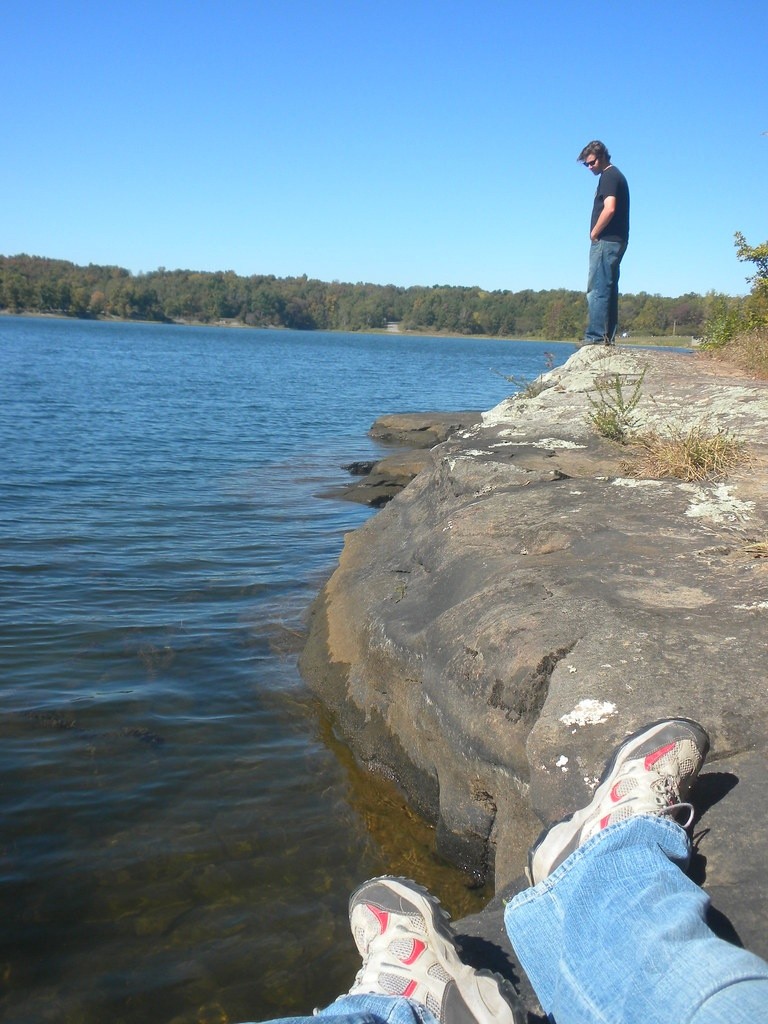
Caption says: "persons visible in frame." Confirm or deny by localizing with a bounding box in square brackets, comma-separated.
[575, 141, 630, 348]
[239, 717, 767, 1024]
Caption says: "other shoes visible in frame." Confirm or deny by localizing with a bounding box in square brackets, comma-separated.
[575, 338, 614, 349]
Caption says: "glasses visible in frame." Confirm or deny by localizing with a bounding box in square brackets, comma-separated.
[584, 155, 600, 167]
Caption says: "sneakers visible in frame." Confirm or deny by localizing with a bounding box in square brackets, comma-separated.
[348, 876, 525, 1024]
[525, 719, 709, 886]
